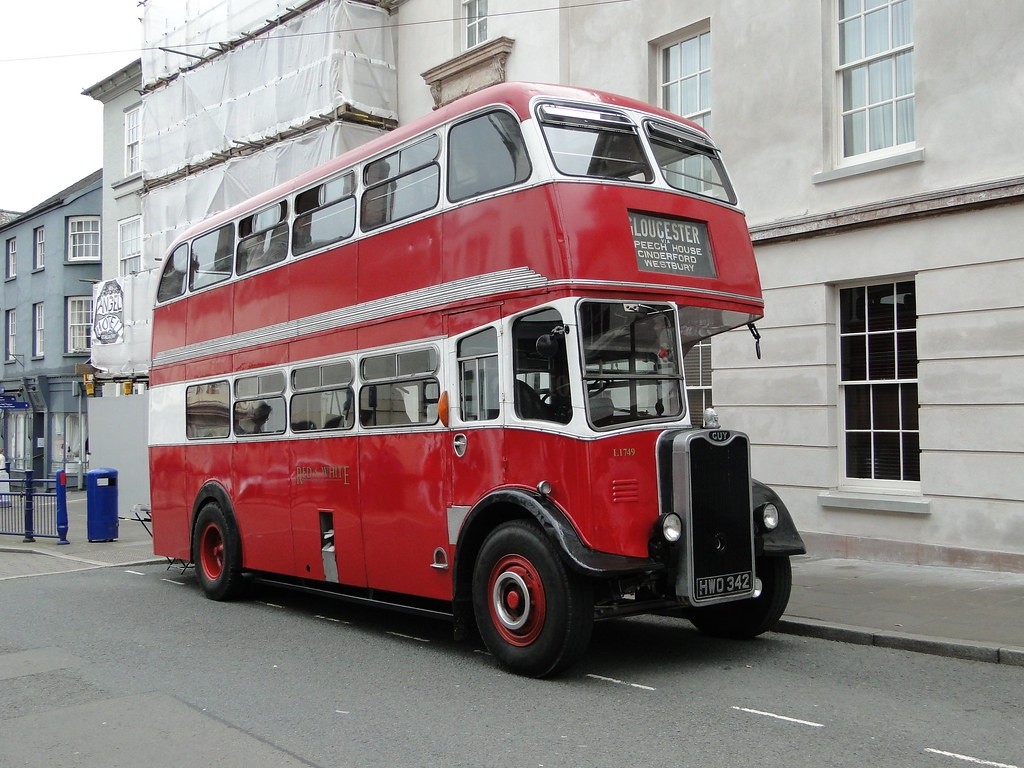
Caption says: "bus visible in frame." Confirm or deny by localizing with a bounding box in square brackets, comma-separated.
[147, 83, 808, 679]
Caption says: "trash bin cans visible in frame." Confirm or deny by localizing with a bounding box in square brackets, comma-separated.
[87, 467, 118, 542]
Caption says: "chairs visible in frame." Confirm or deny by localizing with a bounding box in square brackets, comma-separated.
[517, 378, 552, 420]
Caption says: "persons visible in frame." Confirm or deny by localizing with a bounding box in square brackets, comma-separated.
[240, 401, 272, 432]
[486, 363, 551, 421]
[0, 448, 12, 508]
